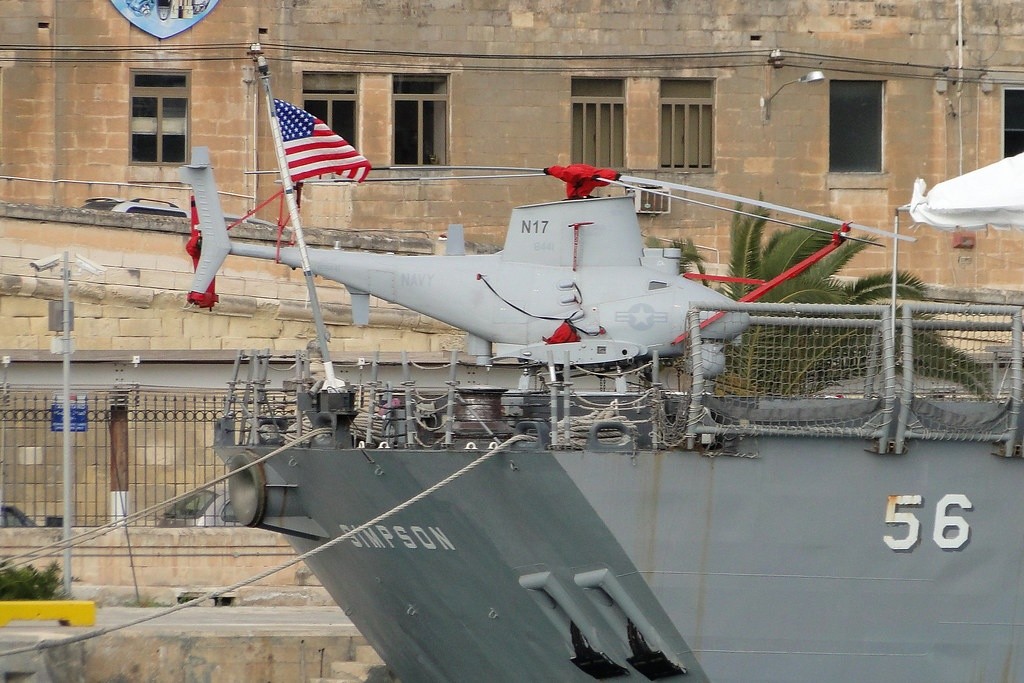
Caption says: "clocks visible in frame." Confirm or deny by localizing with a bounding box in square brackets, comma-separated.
[760, 67, 827, 121]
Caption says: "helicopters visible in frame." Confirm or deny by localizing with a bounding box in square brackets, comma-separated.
[175, 92, 912, 397]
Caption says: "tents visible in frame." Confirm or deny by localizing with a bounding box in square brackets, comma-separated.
[886, 147, 1024, 360]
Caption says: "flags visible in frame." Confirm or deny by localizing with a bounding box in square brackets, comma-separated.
[267, 94, 373, 191]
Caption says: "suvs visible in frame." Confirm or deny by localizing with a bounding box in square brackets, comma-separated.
[79, 195, 191, 218]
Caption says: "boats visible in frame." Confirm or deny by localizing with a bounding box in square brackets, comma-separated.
[209, 325, 1024, 683]
